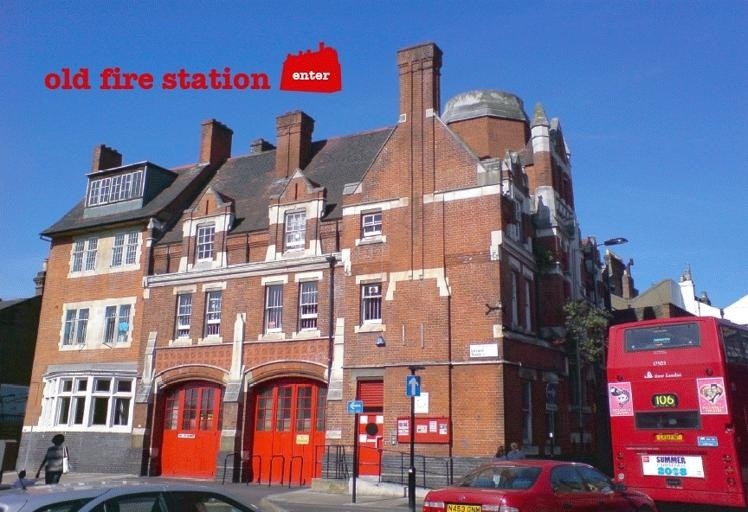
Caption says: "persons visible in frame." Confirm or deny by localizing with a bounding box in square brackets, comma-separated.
[492, 446, 506, 485]
[507, 442, 526, 460]
[36, 433, 69, 484]
[11, 470, 34, 489]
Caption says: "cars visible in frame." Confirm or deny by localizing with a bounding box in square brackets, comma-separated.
[423, 459, 657, 512]
[0, 476, 283, 512]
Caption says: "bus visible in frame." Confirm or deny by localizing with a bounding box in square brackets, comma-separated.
[606, 315, 747, 509]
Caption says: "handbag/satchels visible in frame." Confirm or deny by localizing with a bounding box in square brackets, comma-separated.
[62, 447, 70, 474]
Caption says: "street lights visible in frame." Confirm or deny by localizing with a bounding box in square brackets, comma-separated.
[572, 236, 627, 459]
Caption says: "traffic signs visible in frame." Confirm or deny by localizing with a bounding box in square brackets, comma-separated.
[545, 404, 558, 411]
